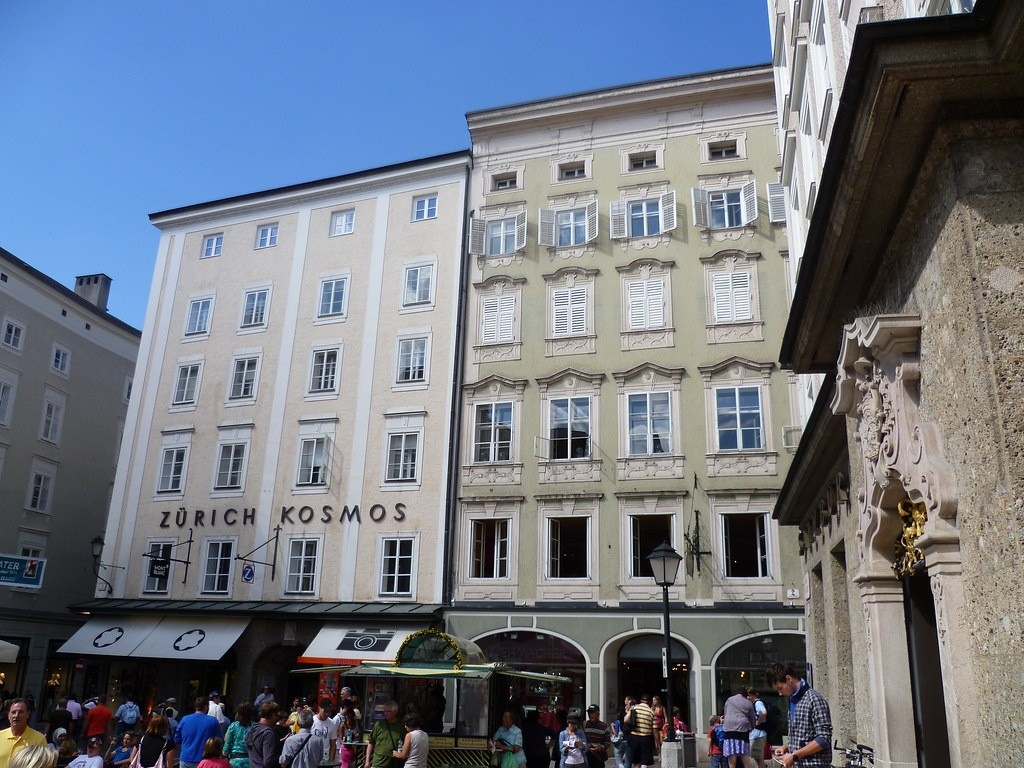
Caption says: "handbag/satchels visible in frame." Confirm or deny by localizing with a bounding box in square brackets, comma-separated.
[609, 720, 624, 745]
[390, 749, 403, 768]
[267, 757, 292, 768]
[500, 746, 519, 768]
[754, 721, 766, 730]
[628, 704, 637, 729]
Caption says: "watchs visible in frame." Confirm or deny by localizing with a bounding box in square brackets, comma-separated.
[793, 752, 799, 762]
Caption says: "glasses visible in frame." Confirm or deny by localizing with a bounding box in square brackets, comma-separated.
[568, 720, 575, 724]
[59, 734, 66, 737]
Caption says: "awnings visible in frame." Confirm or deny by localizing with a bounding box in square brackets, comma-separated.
[295, 621, 432, 666]
[56, 615, 254, 662]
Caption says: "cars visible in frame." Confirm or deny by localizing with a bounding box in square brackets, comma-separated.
[522, 695, 556, 718]
[555, 699, 585, 718]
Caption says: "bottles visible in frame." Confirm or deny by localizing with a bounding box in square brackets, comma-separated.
[347, 728, 350, 742]
[398, 739, 403, 753]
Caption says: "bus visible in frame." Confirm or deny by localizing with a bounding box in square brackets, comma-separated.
[716, 667, 806, 718]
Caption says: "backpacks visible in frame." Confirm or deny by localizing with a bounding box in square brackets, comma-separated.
[130, 735, 168, 768]
[125, 704, 137, 725]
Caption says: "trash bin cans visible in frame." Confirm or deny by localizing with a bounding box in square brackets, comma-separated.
[676, 732, 696, 768]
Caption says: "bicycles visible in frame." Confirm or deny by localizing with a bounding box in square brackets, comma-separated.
[834, 740, 874, 768]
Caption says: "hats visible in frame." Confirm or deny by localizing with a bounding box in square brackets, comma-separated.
[320, 699, 333, 712]
[53, 728, 67, 742]
[566, 712, 578, 724]
[88, 737, 102, 746]
[213, 691, 220, 696]
[587, 704, 599, 712]
[167, 697, 176, 703]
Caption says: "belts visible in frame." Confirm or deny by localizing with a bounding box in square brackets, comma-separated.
[230, 753, 250, 758]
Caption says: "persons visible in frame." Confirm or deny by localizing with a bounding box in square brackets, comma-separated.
[0, 659, 831, 768]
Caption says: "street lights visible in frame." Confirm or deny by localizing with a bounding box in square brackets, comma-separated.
[646, 540, 683, 768]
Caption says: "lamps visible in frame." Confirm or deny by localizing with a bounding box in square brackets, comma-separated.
[89, 535, 125, 595]
